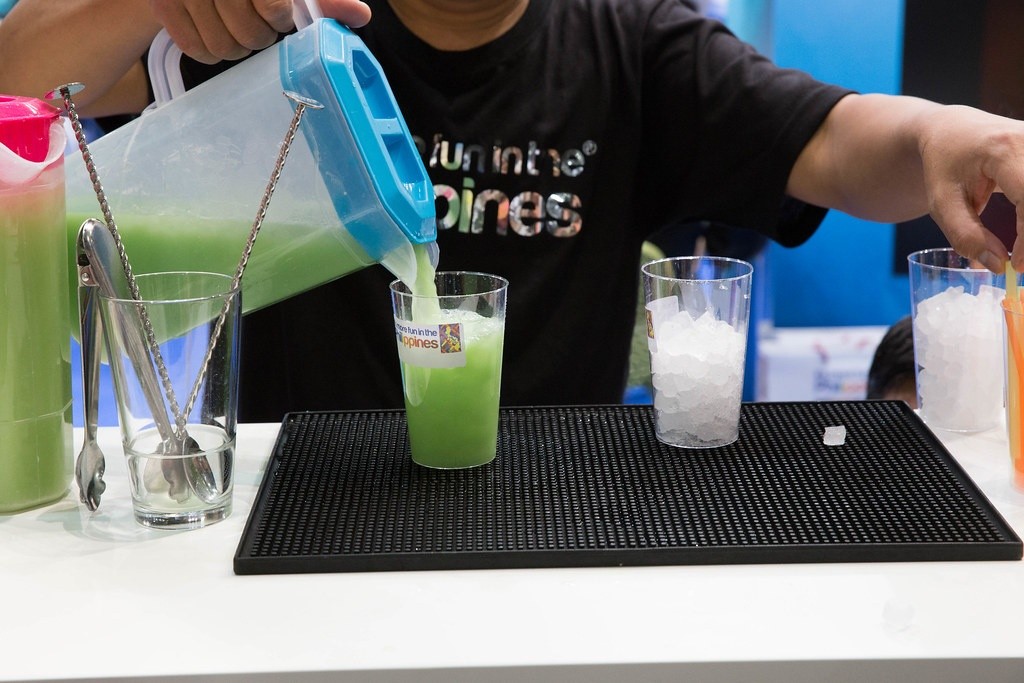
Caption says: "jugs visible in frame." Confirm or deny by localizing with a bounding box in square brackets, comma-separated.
[0, 93, 70, 517]
[64, 1, 439, 369]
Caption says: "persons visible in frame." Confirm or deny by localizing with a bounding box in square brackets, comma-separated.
[0, 0, 1024, 424]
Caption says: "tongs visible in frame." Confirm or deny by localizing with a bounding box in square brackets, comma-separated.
[74, 217, 194, 513]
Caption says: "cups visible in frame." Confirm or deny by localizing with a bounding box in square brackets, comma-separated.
[1000, 285, 1024, 492]
[390, 270, 508, 470]
[906, 247, 1013, 434]
[96, 271, 244, 532]
[640, 255, 754, 450]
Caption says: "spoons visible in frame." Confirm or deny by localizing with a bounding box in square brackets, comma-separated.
[42, 81, 221, 507]
[143, 89, 326, 490]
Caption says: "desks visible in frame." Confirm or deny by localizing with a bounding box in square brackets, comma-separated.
[0, 409, 1024, 683]
